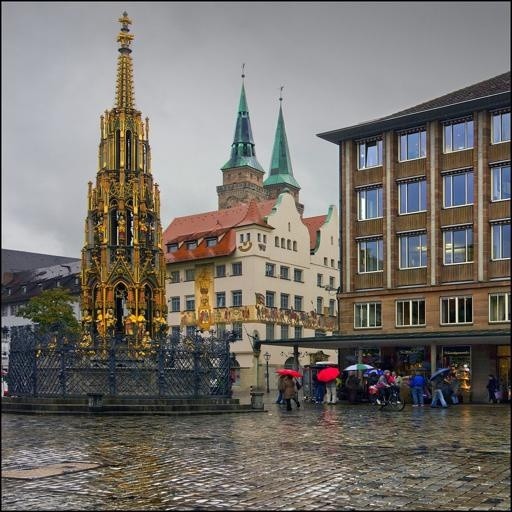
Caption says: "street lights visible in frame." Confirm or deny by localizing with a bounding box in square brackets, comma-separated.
[262, 351, 272, 393]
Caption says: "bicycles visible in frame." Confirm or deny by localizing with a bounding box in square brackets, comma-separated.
[369, 382, 407, 411]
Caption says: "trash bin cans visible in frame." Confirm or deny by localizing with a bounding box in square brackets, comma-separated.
[250, 392, 264, 409]
[87, 392, 104, 412]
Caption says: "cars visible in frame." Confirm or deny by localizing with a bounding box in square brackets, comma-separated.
[1, 369, 32, 397]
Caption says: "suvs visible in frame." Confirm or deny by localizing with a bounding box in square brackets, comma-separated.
[167, 356, 222, 391]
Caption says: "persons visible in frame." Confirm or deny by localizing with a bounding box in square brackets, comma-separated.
[486, 374, 497, 403]
[183, 305, 324, 330]
[49, 306, 165, 357]
[92, 216, 148, 245]
[276, 369, 459, 409]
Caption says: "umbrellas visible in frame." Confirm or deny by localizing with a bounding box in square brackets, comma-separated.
[316, 362, 383, 382]
